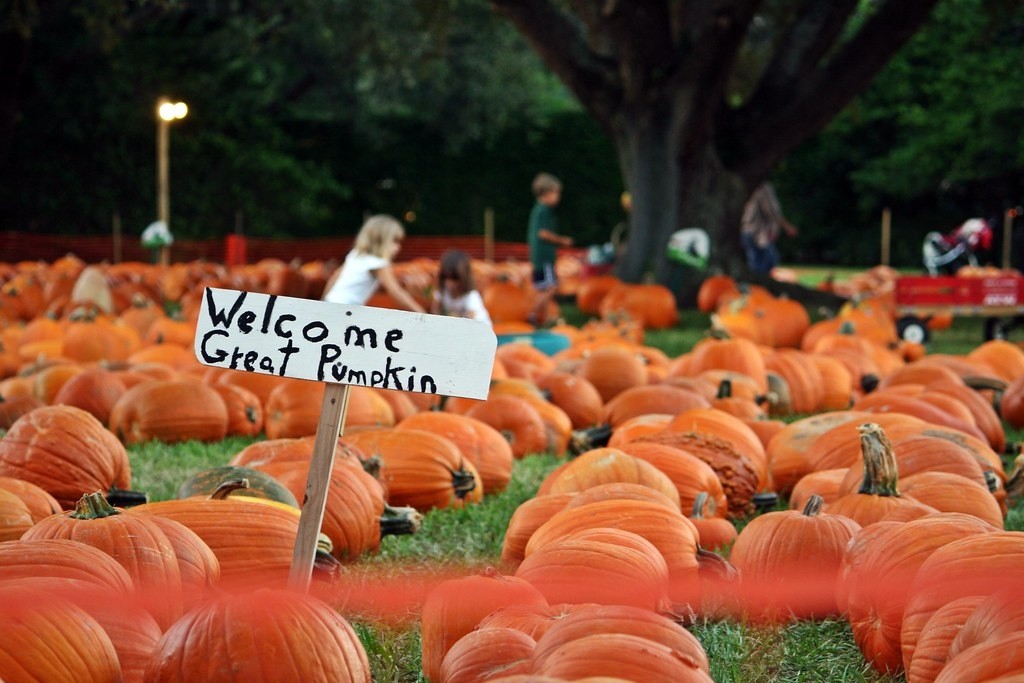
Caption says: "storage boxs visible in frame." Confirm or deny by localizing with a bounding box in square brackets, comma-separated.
[897, 274, 1024, 306]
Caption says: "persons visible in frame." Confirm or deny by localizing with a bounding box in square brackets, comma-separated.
[140, 216, 172, 259]
[528, 174, 573, 291]
[432, 246, 490, 324]
[923, 224, 970, 280]
[663, 218, 710, 299]
[327, 215, 428, 315]
[742, 172, 796, 288]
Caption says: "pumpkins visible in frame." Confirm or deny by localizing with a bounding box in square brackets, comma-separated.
[0, 261, 1024, 683]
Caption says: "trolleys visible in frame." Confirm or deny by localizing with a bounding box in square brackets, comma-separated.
[891, 260, 1024, 345]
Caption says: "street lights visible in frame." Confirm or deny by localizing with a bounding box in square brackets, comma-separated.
[153, 96, 188, 264]
